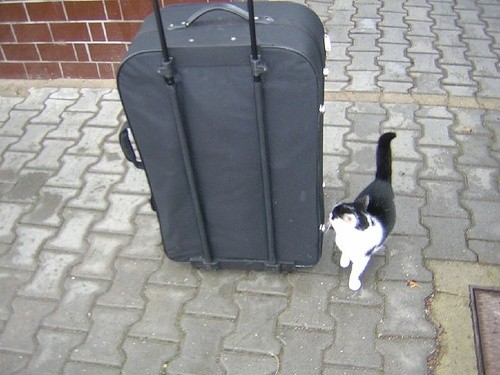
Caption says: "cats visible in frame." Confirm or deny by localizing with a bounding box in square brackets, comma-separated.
[329, 132, 396, 291]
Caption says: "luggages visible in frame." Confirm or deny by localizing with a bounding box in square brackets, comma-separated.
[116, 0, 332, 273]
